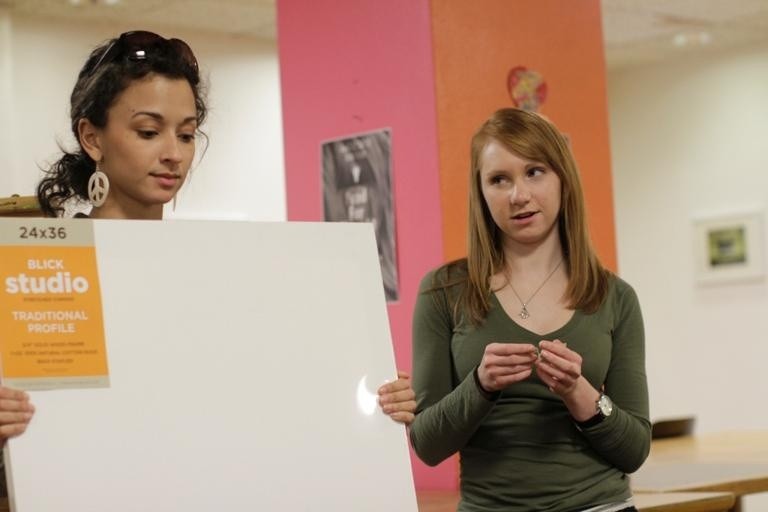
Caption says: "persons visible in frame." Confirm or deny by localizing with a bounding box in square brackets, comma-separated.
[0, 28, 416, 440]
[409, 107, 653, 512]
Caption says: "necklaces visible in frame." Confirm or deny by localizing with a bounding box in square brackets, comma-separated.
[498, 256, 567, 319]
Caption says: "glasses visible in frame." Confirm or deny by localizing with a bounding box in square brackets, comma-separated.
[88, 30, 200, 81]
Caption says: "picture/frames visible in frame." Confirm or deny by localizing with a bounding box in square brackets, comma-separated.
[692, 211, 761, 283]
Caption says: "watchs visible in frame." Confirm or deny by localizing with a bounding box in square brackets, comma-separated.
[569, 388, 613, 433]
